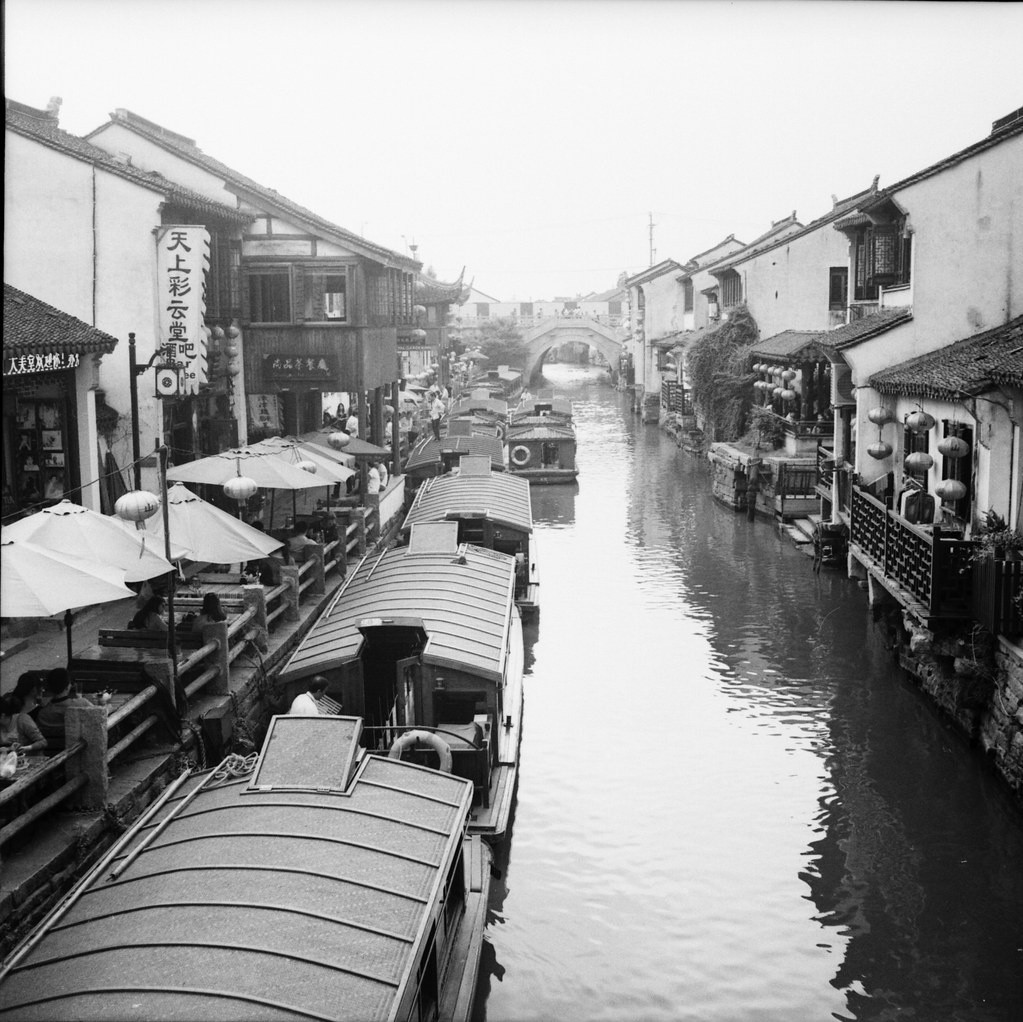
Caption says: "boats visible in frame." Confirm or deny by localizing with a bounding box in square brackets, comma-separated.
[404, 366, 580, 622]
[276, 521, 525, 855]
[0, 715, 492, 1022]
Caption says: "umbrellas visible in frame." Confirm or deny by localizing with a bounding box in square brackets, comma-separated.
[1, 481, 286, 669]
[105, 450, 128, 514]
[156, 426, 388, 521]
[386, 382, 429, 402]
[458, 351, 489, 359]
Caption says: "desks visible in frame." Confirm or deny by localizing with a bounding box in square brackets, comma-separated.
[321, 506, 353, 517]
[4, 755, 49, 781]
[74, 692, 136, 716]
[914, 522, 963, 541]
[72, 644, 198, 666]
[159, 611, 244, 626]
[176, 583, 275, 600]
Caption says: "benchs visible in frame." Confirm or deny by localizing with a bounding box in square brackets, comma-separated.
[67, 658, 154, 695]
[198, 572, 240, 587]
[162, 597, 244, 646]
[97, 629, 202, 676]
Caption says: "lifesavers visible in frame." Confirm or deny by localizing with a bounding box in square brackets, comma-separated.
[387, 729, 453, 775]
[511, 445, 531, 466]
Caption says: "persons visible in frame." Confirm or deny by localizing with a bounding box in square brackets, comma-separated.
[20, 405, 59, 466]
[1, 672, 96, 815]
[130, 364, 470, 633]
[511, 307, 600, 322]
[763, 390, 834, 433]
[291, 676, 328, 716]
[1, 476, 63, 516]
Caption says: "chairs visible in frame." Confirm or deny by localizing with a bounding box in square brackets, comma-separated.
[905, 489, 936, 525]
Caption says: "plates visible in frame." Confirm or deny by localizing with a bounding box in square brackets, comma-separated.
[16, 762, 29, 770]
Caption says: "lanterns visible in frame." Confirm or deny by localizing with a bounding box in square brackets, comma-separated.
[114, 432, 350, 531]
[867, 406, 968, 501]
[403, 352, 456, 380]
[412, 281, 463, 341]
[621, 290, 676, 370]
[211, 325, 240, 376]
[753, 363, 796, 400]
